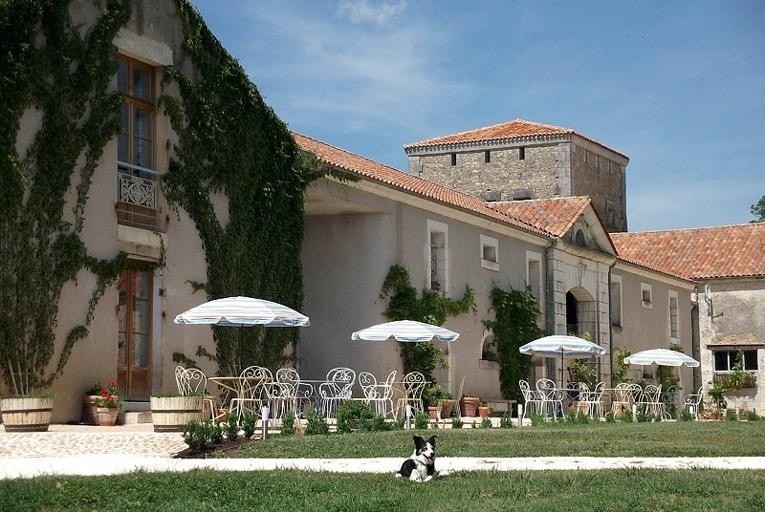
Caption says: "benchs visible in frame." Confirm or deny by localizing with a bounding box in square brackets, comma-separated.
[483, 399, 517, 418]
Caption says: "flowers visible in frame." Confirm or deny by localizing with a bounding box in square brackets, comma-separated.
[96, 394, 122, 410]
[86, 379, 121, 396]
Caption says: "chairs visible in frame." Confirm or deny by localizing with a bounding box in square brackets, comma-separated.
[176, 366, 426, 426]
[518, 378, 705, 421]
[437, 375, 465, 421]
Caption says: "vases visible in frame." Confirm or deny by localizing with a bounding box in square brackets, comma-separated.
[84, 395, 119, 424]
[97, 408, 120, 426]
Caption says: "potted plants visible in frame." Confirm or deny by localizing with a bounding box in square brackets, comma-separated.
[150, 392, 204, 432]
[427, 383, 492, 419]
[0, 391, 57, 433]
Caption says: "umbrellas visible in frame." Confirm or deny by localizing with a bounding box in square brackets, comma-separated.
[623, 348, 701, 392]
[519, 335, 607, 388]
[351, 320, 462, 381]
[174, 295, 311, 375]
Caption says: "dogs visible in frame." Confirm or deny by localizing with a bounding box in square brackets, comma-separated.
[393, 433, 449, 485]
